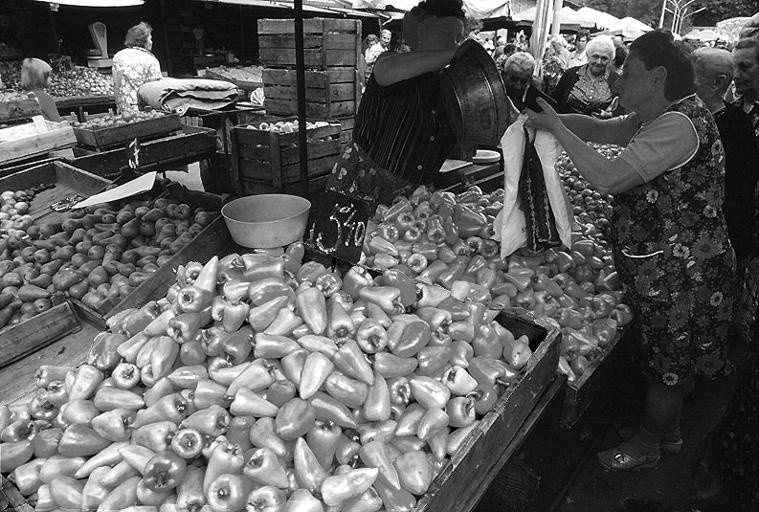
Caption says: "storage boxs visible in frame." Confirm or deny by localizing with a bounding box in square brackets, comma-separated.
[233, 14, 365, 201]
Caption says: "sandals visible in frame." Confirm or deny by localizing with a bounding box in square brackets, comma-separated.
[597, 438, 684, 473]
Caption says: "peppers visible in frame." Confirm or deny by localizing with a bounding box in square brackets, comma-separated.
[0, 185, 633, 512]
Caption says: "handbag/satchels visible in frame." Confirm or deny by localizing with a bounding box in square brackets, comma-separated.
[517, 123, 563, 255]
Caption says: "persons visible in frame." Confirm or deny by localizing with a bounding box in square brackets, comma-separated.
[678, 34, 758, 354]
[110, 19, 164, 116]
[525, 30, 735, 472]
[304, 2, 628, 277]
[18, 56, 62, 122]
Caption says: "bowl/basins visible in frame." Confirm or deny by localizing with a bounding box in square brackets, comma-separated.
[438, 38, 508, 149]
[222, 193, 311, 249]
